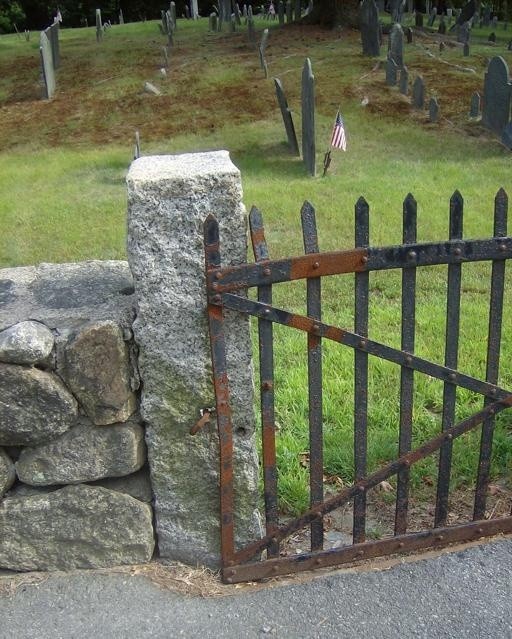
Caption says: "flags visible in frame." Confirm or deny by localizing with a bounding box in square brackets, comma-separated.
[331, 113, 346, 151]
